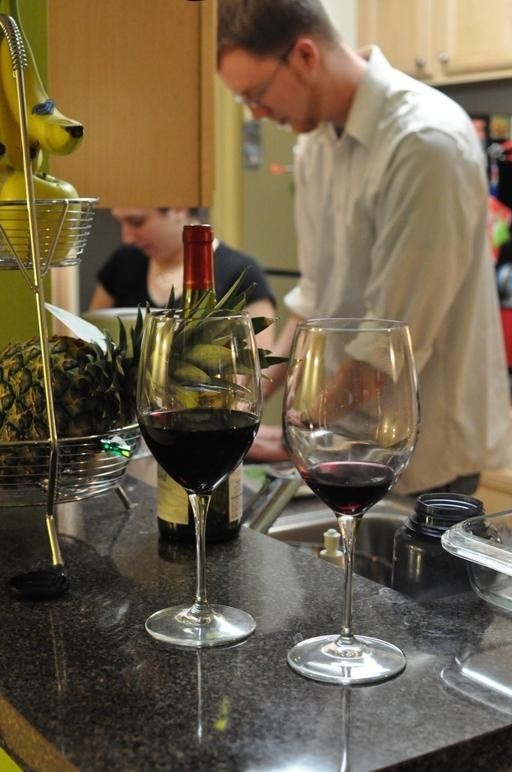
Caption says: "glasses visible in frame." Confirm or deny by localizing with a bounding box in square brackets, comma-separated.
[235, 61, 287, 107]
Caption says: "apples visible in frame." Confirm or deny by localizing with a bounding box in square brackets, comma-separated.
[0, 169, 82, 264]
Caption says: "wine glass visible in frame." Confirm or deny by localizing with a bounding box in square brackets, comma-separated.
[134, 306, 267, 647]
[279, 316, 421, 687]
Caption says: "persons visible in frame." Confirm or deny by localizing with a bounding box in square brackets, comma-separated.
[176, 0, 511, 521]
[87, 206, 278, 398]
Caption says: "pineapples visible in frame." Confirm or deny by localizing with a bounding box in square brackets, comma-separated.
[0, 266, 292, 491]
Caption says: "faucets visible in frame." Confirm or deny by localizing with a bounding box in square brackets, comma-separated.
[238, 462, 309, 532]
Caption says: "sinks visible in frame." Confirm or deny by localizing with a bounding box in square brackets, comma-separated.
[242, 500, 510, 599]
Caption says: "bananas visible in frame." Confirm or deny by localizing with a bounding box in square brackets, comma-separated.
[0, 19, 84, 177]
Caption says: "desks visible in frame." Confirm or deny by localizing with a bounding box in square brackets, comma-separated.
[0, 474, 512, 772]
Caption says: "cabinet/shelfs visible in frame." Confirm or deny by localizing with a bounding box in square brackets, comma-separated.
[354, 0, 512, 88]
[47, 0, 216, 206]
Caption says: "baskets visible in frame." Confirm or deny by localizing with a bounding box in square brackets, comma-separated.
[0, 423, 141, 506]
[0, 197, 97, 271]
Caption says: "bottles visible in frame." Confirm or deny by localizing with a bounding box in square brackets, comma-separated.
[392, 489, 504, 644]
[151, 219, 245, 546]
